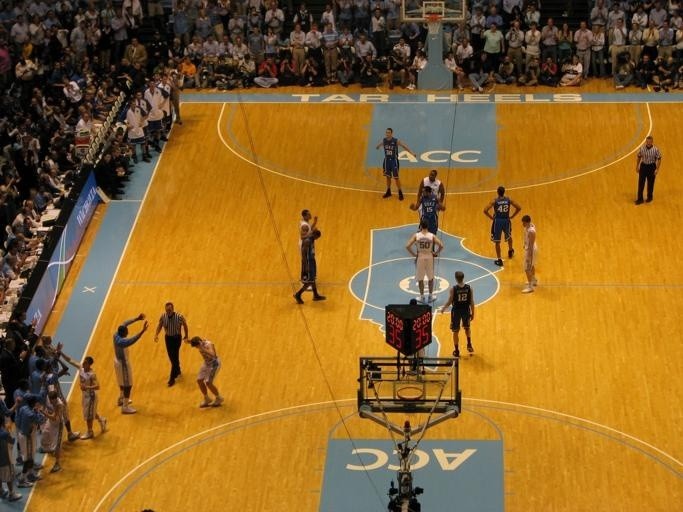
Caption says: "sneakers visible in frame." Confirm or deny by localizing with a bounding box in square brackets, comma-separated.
[168, 378, 175, 385]
[427, 295, 437, 303]
[16, 479, 34, 489]
[8, 492, 22, 501]
[526, 280, 537, 286]
[117, 397, 132, 406]
[520, 287, 534, 293]
[383, 192, 391, 198]
[398, 192, 404, 200]
[467, 344, 473, 353]
[199, 397, 212, 408]
[494, 259, 503, 267]
[67, 431, 81, 441]
[207, 396, 224, 406]
[312, 295, 326, 301]
[507, 248, 515, 258]
[100, 418, 106, 433]
[50, 463, 63, 474]
[80, 431, 94, 439]
[452, 350, 459, 356]
[122, 406, 137, 414]
[292, 293, 305, 305]
[414, 297, 425, 303]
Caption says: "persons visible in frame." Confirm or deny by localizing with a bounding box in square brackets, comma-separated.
[440, 271, 477, 356]
[64, 355, 107, 439]
[419, 171, 444, 212]
[405, 218, 444, 304]
[410, 185, 444, 236]
[483, 187, 521, 266]
[112, 314, 149, 415]
[374, 128, 417, 201]
[154, 304, 189, 386]
[520, 215, 537, 293]
[442, 1, 683, 91]
[189, 336, 223, 409]
[0, 0, 181, 202]
[2, 202, 79, 503]
[407, 298, 425, 373]
[292, 230, 326, 304]
[636, 136, 662, 206]
[171, 1, 427, 92]
[300, 210, 316, 244]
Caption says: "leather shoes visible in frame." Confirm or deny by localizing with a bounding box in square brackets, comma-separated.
[646, 198, 652, 202]
[634, 199, 644, 205]
